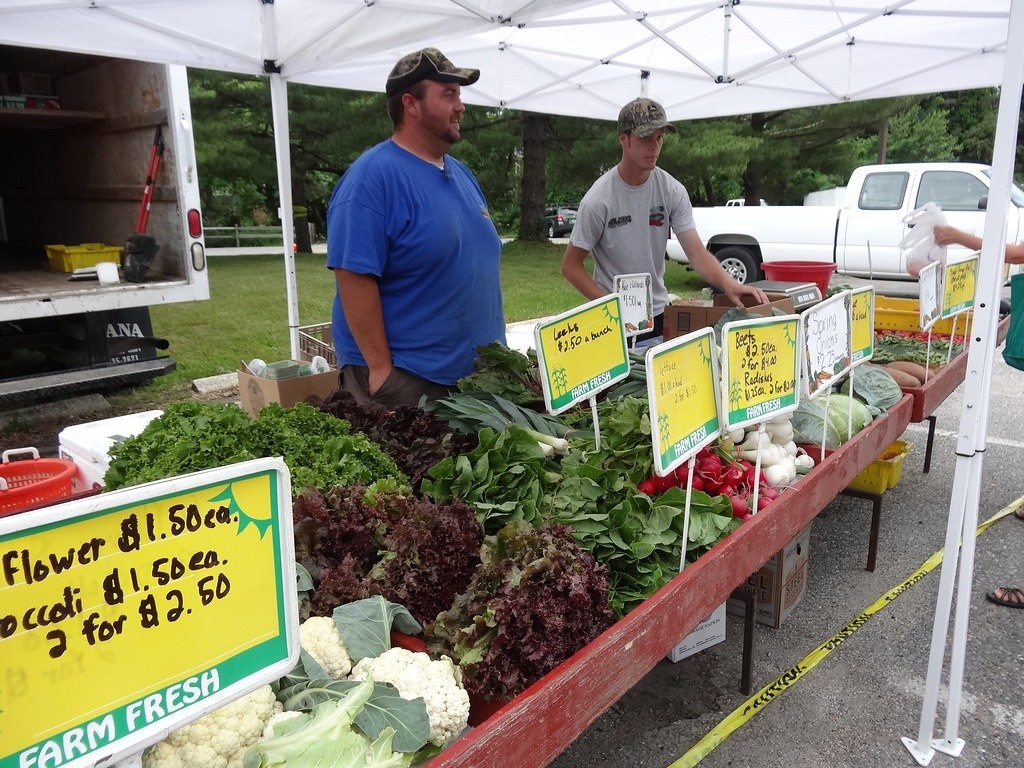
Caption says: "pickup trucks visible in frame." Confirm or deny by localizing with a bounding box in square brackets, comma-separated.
[726, 198, 767, 208]
[660, 163, 1024, 298]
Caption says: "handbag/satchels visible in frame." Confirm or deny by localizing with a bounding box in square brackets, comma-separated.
[899, 201, 948, 276]
[1001, 273, 1024, 371]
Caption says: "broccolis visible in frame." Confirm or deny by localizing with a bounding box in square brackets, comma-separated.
[138, 614, 469, 768]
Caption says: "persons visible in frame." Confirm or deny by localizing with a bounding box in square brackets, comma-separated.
[933, 226, 1024, 609]
[561, 99, 770, 348]
[323, 47, 508, 409]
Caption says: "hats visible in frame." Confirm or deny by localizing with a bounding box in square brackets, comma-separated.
[386, 47, 481, 97]
[618, 97, 677, 138]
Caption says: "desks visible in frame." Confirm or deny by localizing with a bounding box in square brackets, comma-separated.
[424, 306, 1015, 767]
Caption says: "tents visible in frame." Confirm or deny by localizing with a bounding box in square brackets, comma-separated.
[0, 0, 1024, 768]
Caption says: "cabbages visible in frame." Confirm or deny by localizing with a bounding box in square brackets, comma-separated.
[790, 363, 904, 450]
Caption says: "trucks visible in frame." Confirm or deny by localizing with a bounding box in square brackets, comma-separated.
[0, 44, 216, 410]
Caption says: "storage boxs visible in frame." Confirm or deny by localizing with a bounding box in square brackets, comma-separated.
[663, 292, 794, 350]
[56, 406, 166, 494]
[667, 601, 725, 664]
[847, 439, 911, 494]
[727, 519, 815, 627]
[43, 242, 124, 272]
[0, 71, 61, 110]
[236, 319, 334, 415]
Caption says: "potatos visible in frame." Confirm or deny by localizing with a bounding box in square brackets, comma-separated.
[880, 361, 935, 387]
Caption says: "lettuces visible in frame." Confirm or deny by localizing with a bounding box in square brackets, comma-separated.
[100, 396, 615, 700]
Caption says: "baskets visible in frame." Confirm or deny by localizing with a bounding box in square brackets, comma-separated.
[0, 447, 76, 516]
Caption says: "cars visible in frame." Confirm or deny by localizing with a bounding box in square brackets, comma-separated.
[545, 206, 579, 239]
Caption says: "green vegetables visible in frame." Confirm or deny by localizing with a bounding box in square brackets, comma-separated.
[302, 339, 749, 619]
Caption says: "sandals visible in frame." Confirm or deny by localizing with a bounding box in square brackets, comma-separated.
[1015, 507, 1024, 519]
[987, 585, 1024, 608]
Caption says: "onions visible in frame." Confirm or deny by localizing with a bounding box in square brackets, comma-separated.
[724, 410, 815, 492]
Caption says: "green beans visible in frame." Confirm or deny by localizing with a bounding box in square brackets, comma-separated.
[870, 334, 964, 364]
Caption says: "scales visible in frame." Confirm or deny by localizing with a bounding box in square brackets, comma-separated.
[741, 280, 823, 312]
